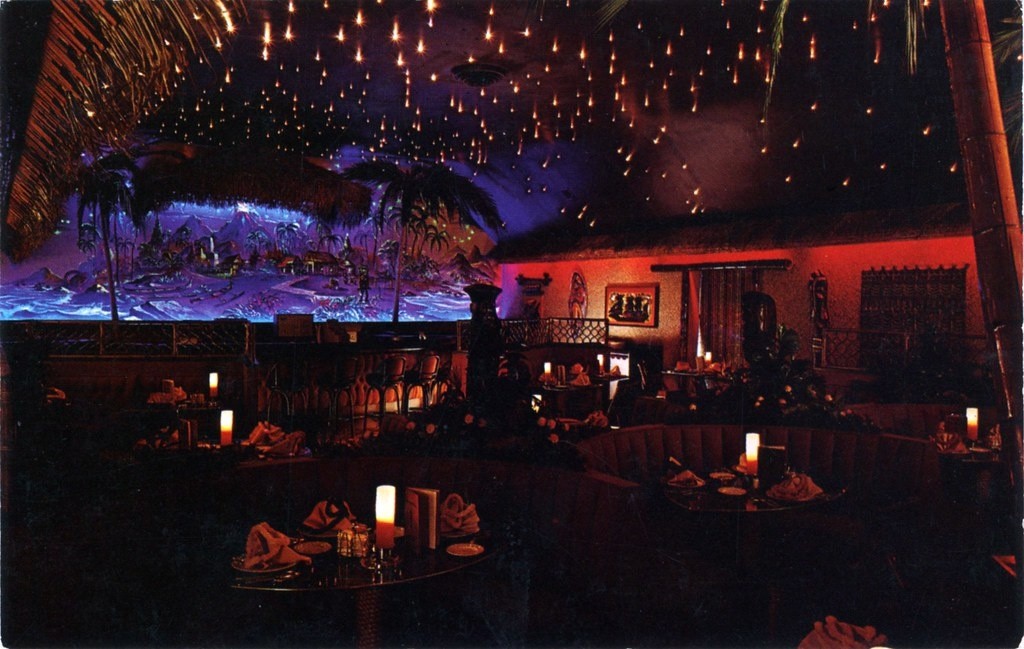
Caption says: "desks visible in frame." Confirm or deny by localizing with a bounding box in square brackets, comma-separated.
[662, 370, 716, 413]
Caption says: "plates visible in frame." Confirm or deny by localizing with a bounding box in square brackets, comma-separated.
[232, 553, 296, 572]
[447, 543, 483, 556]
[718, 487, 746, 495]
[440, 530, 479, 538]
[798, 624, 890, 649]
[767, 489, 816, 501]
[296, 525, 337, 537]
[936, 449, 969, 454]
[667, 479, 705, 487]
[709, 472, 735, 480]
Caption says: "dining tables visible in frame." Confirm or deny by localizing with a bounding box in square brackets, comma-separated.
[660, 472, 851, 648]
[956, 455, 1004, 506]
[221, 531, 505, 649]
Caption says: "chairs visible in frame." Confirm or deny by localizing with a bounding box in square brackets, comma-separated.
[266, 352, 465, 439]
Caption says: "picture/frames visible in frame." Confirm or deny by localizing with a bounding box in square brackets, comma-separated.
[605, 286, 658, 329]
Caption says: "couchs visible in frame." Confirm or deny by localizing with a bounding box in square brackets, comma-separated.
[840, 402, 1005, 530]
[567, 425, 942, 629]
[232, 456, 649, 648]
